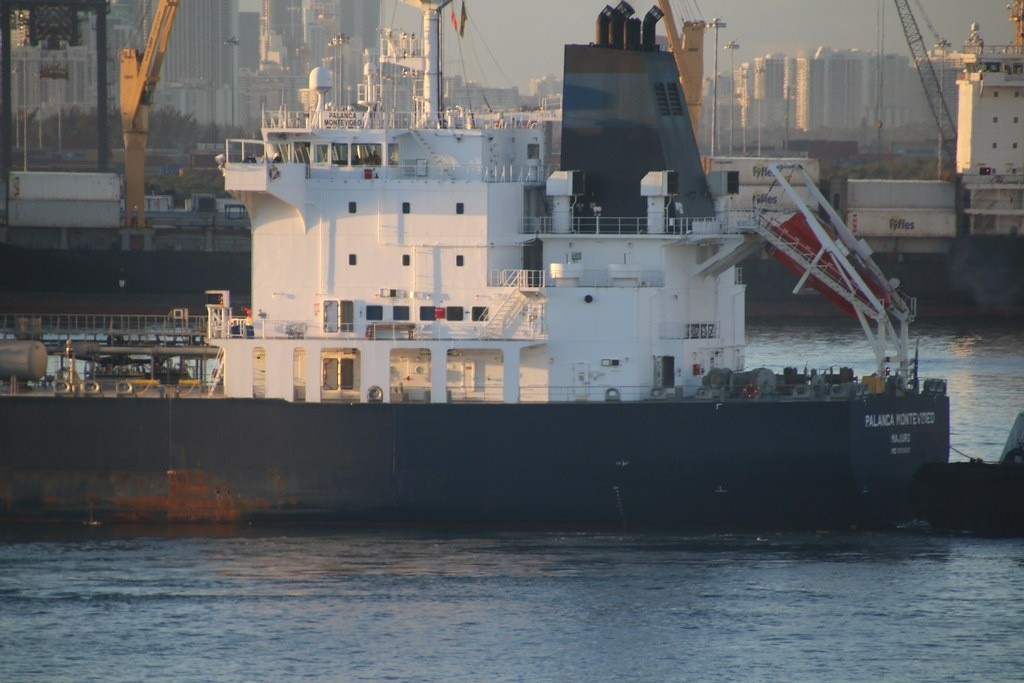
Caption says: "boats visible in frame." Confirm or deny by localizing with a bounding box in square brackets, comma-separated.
[0, 0, 949, 536]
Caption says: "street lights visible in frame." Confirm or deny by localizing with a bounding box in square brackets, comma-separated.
[327, 33, 349, 110]
[722, 39, 739, 157]
[704, 17, 727, 157]
[738, 57, 766, 156]
[224, 36, 239, 139]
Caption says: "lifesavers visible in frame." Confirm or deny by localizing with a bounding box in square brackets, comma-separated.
[742, 383, 758, 398]
